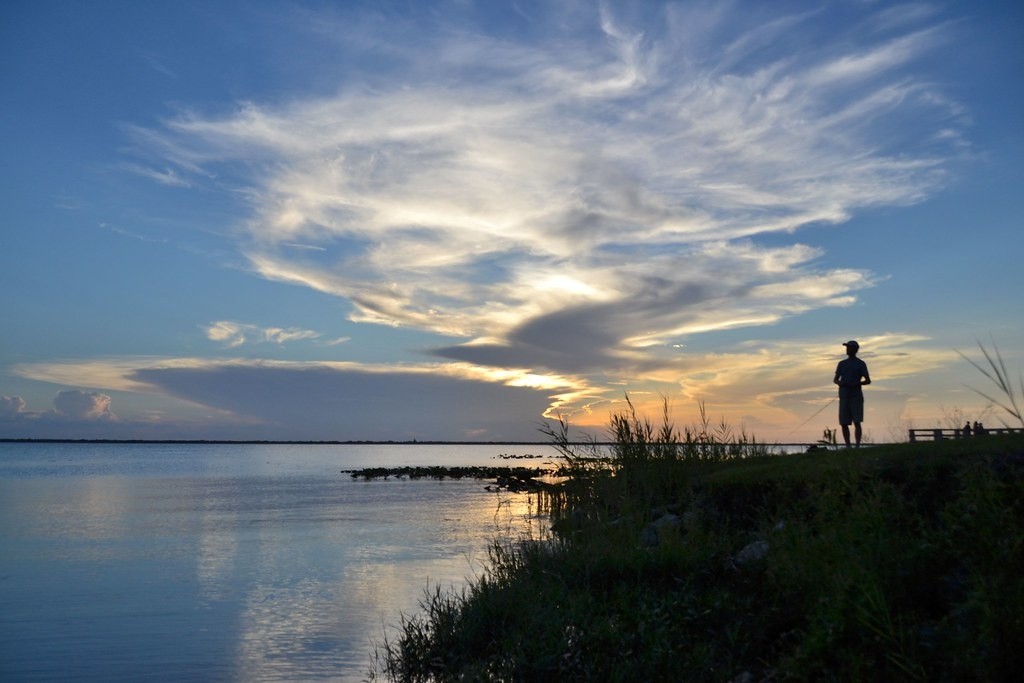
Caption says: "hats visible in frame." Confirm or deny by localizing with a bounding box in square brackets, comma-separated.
[843, 341, 859, 348]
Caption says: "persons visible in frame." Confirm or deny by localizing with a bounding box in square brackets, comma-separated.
[834, 340, 872, 446]
[964, 421, 971, 436]
[974, 421, 983, 435]
